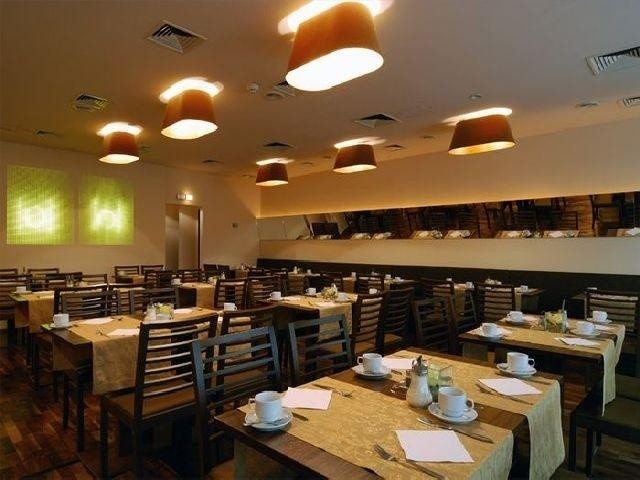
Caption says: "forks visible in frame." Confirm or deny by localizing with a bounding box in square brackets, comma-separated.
[372, 442, 447, 480]
[310, 382, 354, 398]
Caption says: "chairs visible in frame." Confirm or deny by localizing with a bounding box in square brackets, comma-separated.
[1, 261, 640, 480]
[310, 192, 638, 241]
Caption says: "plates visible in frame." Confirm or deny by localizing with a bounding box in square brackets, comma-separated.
[424, 402, 480, 424]
[245, 409, 291, 432]
[9, 291, 32, 294]
[478, 333, 502, 340]
[49, 323, 72, 329]
[496, 361, 538, 378]
[171, 283, 183, 286]
[351, 363, 392, 380]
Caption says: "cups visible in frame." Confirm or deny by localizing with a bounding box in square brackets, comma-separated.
[16, 286, 27, 293]
[438, 386, 474, 418]
[357, 352, 383, 372]
[248, 390, 282, 424]
[53, 314, 69, 326]
[384, 274, 528, 291]
[223, 302, 237, 311]
[174, 279, 180, 284]
[480, 310, 608, 333]
[271, 287, 348, 301]
[506, 352, 535, 371]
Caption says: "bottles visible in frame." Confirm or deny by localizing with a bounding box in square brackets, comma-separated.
[147, 304, 156, 321]
[405, 356, 433, 407]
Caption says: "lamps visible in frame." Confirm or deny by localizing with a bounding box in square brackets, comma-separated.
[157, 75, 225, 141]
[251, 155, 296, 188]
[95, 121, 146, 166]
[276, 0, 395, 94]
[441, 106, 518, 157]
[331, 133, 388, 178]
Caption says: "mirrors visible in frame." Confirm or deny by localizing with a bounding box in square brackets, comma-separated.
[256, 191, 640, 242]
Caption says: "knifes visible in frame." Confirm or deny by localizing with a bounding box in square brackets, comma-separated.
[416, 416, 493, 445]
